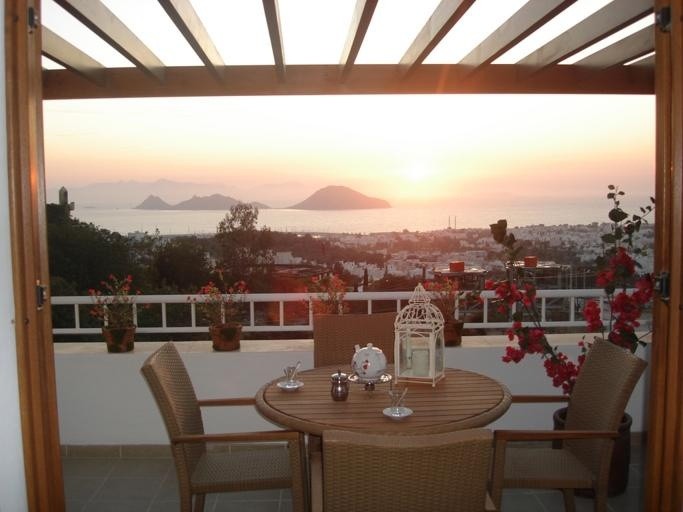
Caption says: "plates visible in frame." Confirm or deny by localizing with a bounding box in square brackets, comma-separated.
[276, 380, 304, 391]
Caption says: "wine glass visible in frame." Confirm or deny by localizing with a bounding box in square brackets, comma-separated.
[387, 386, 408, 415]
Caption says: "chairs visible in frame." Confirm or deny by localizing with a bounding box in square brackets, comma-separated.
[141, 342, 307, 512]
[487, 337, 648, 511]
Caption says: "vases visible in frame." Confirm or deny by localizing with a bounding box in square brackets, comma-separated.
[209, 325, 242, 351]
[444, 321, 464, 347]
[553, 408, 632, 497]
[101, 325, 136, 353]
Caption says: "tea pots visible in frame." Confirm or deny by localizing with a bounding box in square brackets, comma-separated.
[352, 343, 386, 378]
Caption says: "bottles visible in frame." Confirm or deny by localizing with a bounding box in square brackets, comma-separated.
[330, 367, 350, 401]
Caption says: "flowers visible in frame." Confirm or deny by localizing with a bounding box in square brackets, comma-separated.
[86, 273, 151, 327]
[484, 186, 656, 401]
[299, 270, 350, 315]
[186, 280, 251, 325]
[423, 270, 473, 321]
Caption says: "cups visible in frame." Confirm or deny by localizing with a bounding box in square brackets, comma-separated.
[284, 366, 297, 386]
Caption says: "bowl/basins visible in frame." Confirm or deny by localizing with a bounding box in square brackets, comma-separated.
[382, 406, 413, 420]
